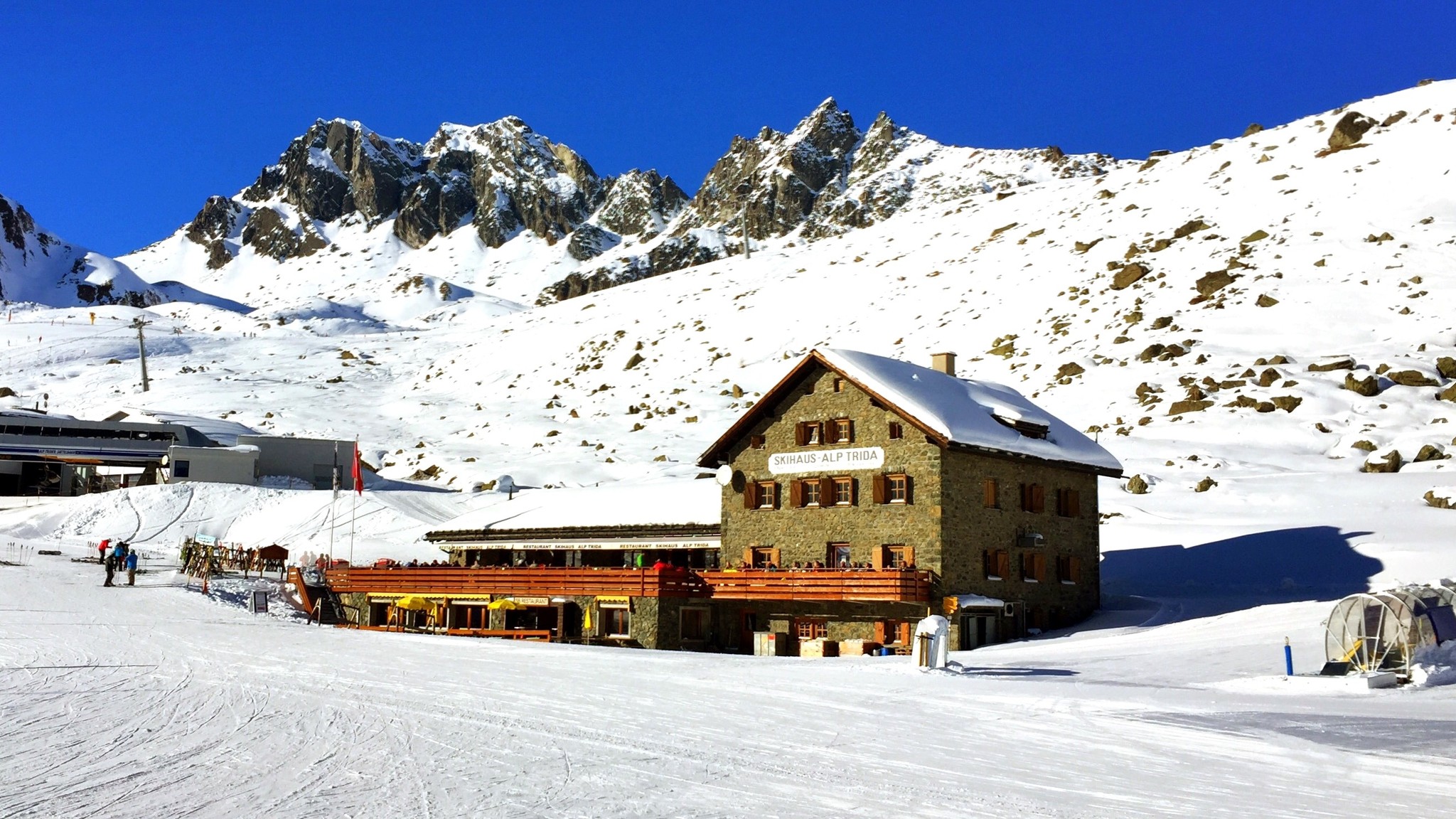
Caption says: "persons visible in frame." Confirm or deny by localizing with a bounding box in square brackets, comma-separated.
[789, 560, 822, 572]
[637, 551, 684, 571]
[299, 551, 309, 566]
[112, 541, 129, 572]
[566, 564, 629, 570]
[724, 561, 777, 572]
[372, 559, 461, 569]
[471, 560, 480, 570]
[125, 549, 138, 586]
[501, 559, 552, 570]
[885, 561, 919, 573]
[316, 553, 326, 570]
[839, 561, 876, 572]
[103, 552, 116, 587]
[98, 538, 112, 564]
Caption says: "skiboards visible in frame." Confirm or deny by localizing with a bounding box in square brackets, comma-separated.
[0, 536, 256, 588]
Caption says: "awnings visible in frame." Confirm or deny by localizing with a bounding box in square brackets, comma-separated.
[439, 536, 722, 555]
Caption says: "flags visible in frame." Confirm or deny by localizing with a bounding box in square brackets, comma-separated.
[351, 442, 363, 495]
[333, 450, 339, 499]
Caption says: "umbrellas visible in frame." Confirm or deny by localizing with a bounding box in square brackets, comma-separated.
[396, 596, 435, 612]
[487, 599, 526, 611]
[584, 602, 594, 644]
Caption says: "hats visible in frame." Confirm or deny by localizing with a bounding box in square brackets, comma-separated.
[108, 538, 112, 542]
[130, 549, 135, 554]
